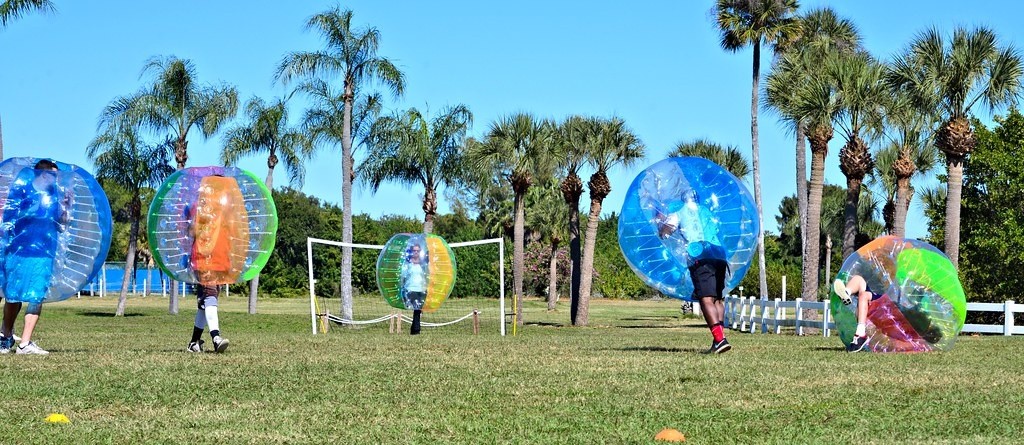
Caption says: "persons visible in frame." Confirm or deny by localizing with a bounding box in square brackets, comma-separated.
[400, 244, 430, 335]
[834, 252, 946, 353]
[0, 160, 76, 355]
[656, 189, 732, 355]
[183, 174, 229, 354]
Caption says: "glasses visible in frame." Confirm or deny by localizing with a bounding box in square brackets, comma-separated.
[46, 171, 57, 178]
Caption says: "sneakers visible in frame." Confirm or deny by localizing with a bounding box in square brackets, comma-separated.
[16, 341, 50, 356]
[213, 335, 230, 354]
[187, 340, 203, 353]
[0, 333, 15, 354]
[834, 279, 852, 305]
[848, 334, 869, 354]
[707, 338, 731, 354]
